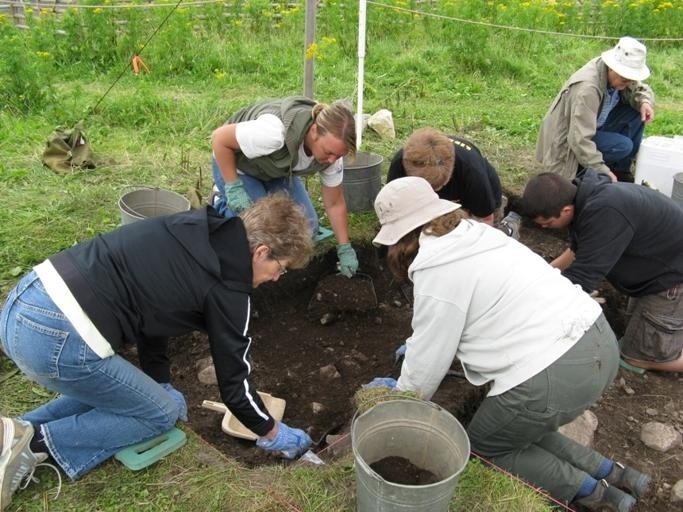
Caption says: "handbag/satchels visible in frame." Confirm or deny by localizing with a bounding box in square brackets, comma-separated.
[44, 119, 105, 178]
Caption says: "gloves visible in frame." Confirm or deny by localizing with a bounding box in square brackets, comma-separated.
[394, 343, 407, 362]
[364, 377, 396, 391]
[257, 420, 313, 460]
[223, 180, 255, 216]
[335, 242, 360, 278]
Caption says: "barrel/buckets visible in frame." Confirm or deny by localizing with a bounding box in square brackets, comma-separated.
[633, 134, 683, 198]
[117, 184, 192, 226]
[670, 172, 683, 204]
[350, 394, 472, 512]
[339, 150, 384, 212]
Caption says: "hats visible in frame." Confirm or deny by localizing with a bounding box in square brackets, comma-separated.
[372, 176, 463, 249]
[601, 36, 651, 82]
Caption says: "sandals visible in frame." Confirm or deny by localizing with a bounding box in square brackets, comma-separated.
[207, 181, 222, 205]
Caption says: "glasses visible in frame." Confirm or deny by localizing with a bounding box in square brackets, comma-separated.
[273, 256, 288, 276]
[620, 77, 632, 83]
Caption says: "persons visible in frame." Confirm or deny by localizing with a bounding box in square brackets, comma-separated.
[370, 176, 652, 511]
[211, 97, 359, 280]
[534, 37, 655, 183]
[522, 172, 683, 371]
[377, 125, 524, 269]
[0, 191, 313, 511]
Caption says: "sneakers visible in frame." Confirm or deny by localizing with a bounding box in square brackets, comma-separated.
[1, 417, 61, 512]
[604, 460, 653, 497]
[572, 479, 636, 512]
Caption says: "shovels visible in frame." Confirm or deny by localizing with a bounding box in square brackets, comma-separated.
[271, 422, 345, 456]
